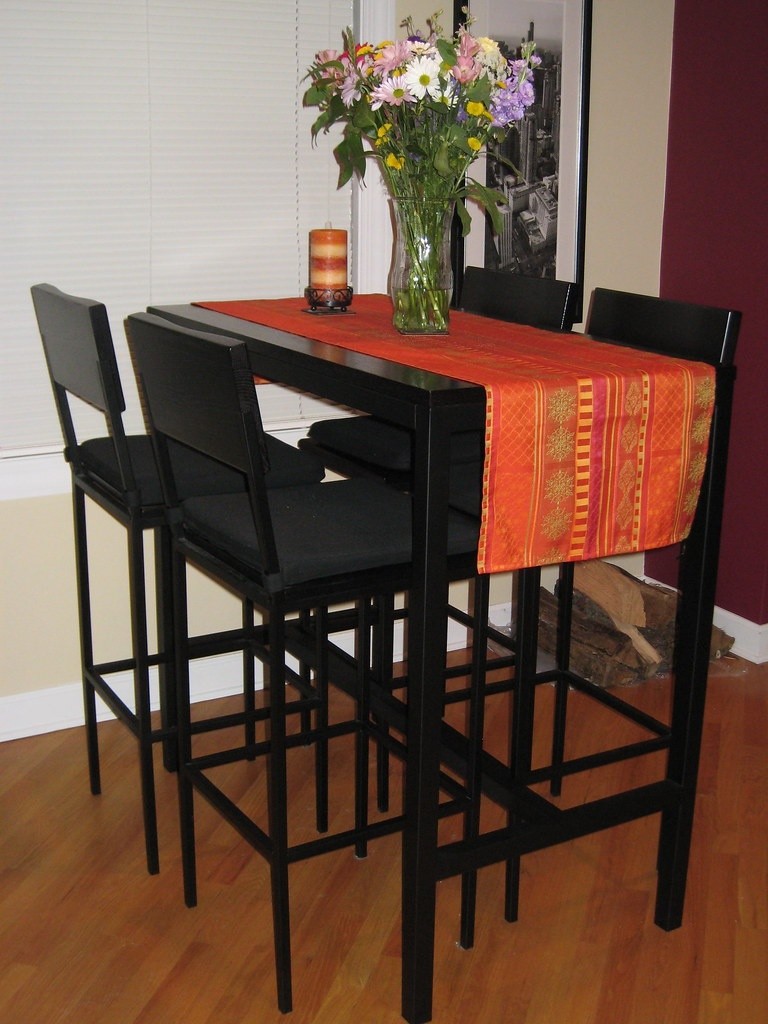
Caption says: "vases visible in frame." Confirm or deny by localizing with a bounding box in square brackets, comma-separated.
[388, 194, 453, 334]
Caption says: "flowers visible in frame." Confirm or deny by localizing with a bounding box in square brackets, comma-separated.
[301, 6, 541, 213]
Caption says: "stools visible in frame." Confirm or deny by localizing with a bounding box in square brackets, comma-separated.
[30, 266, 744, 1024]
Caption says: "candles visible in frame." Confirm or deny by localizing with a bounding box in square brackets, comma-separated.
[309, 220, 348, 303]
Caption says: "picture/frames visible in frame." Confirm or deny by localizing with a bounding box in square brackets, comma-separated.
[452, 0, 593, 324]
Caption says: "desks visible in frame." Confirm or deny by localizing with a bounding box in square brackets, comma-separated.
[148, 291, 736, 1023]
